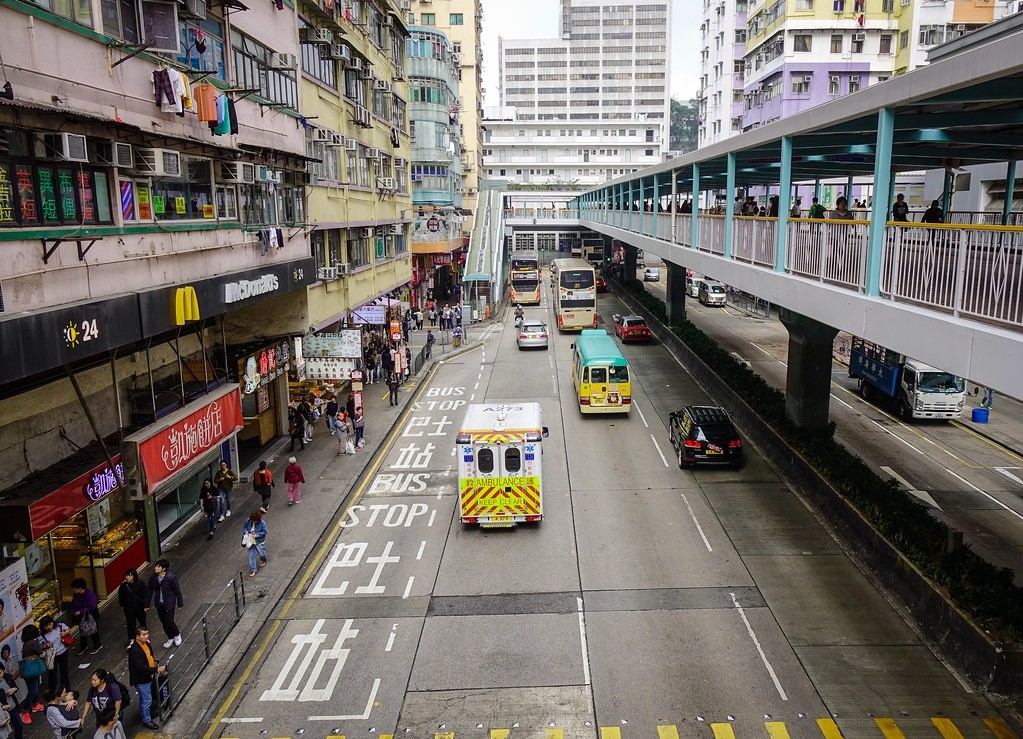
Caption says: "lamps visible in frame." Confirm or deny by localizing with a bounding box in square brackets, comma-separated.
[271, 50, 299, 72]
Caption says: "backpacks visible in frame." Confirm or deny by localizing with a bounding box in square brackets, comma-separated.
[814, 204, 824, 219]
[106, 682, 131, 711]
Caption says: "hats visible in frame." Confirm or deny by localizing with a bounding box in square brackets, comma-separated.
[302, 396, 308, 401]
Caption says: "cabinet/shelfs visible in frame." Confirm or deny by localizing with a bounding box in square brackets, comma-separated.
[32, 591, 60, 632]
[41, 512, 88, 553]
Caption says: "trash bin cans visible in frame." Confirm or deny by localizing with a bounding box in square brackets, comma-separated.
[452, 335, 461, 346]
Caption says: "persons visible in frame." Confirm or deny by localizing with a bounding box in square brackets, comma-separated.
[199, 457, 305, 542]
[242, 510, 268, 577]
[980, 386, 994, 409]
[364, 281, 525, 406]
[893, 193, 909, 239]
[0, 643, 21, 678]
[288, 395, 365, 455]
[921, 200, 944, 247]
[0, 559, 185, 739]
[609, 188, 867, 274]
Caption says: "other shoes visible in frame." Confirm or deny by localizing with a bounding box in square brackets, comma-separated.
[987, 406, 992, 409]
[395, 402, 398, 406]
[366, 375, 410, 384]
[250, 572, 256, 576]
[980, 403, 984, 407]
[260, 561, 266, 567]
[287, 430, 363, 456]
[390, 403, 394, 406]
[260, 500, 302, 513]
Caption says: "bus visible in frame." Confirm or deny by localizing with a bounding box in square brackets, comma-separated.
[549, 258, 597, 331]
[509, 250, 542, 306]
[580, 238, 645, 268]
[570, 329, 631, 414]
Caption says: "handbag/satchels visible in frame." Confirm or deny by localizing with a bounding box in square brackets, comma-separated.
[346, 438, 355, 455]
[44, 647, 56, 670]
[18, 655, 47, 679]
[80, 609, 97, 635]
[146, 601, 156, 614]
[288, 423, 297, 434]
[63, 634, 73, 644]
[405, 368, 409, 375]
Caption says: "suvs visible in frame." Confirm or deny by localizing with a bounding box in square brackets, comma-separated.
[669, 405, 745, 469]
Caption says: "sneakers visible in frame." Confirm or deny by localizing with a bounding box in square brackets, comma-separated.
[31, 703, 45, 713]
[174, 633, 182, 646]
[90, 644, 103, 654]
[75, 645, 89, 655]
[20, 711, 32, 724]
[208, 510, 230, 536]
[163, 639, 174, 649]
[145, 721, 159, 729]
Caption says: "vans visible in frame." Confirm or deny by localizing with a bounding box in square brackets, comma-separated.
[686, 277, 704, 297]
[697, 280, 727, 308]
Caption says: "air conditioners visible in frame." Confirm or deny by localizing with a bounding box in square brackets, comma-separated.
[95, 142, 135, 171]
[299, 168, 321, 188]
[446, 42, 462, 75]
[138, 146, 182, 180]
[179, 0, 208, 23]
[313, 128, 332, 142]
[353, 103, 375, 129]
[927, 24, 965, 31]
[389, 224, 405, 235]
[325, 133, 345, 146]
[455, 139, 476, 195]
[364, 148, 423, 191]
[318, 266, 338, 281]
[32, 130, 91, 164]
[215, 159, 255, 188]
[732, 10, 866, 126]
[336, 262, 352, 275]
[309, 13, 406, 94]
[362, 227, 376, 239]
[347, 139, 358, 151]
[256, 162, 270, 182]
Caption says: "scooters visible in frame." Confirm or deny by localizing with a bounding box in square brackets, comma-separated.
[514, 317, 522, 327]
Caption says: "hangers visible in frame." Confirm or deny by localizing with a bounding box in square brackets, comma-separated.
[155, 58, 170, 70]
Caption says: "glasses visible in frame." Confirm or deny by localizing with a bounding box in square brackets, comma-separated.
[113, 715, 119, 720]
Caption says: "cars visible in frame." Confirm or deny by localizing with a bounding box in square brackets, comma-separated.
[615, 313, 648, 343]
[595, 276, 607, 292]
[620, 260, 647, 269]
[515, 318, 548, 350]
[643, 268, 660, 281]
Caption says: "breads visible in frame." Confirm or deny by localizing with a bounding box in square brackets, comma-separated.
[90, 521, 138, 556]
[31, 592, 58, 627]
[38, 516, 85, 549]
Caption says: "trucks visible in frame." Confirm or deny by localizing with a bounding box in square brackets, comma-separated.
[847, 335, 966, 422]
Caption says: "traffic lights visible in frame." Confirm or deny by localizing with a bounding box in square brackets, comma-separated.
[605, 253, 611, 264]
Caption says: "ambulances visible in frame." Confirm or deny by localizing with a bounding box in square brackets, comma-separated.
[455, 402, 549, 530]
